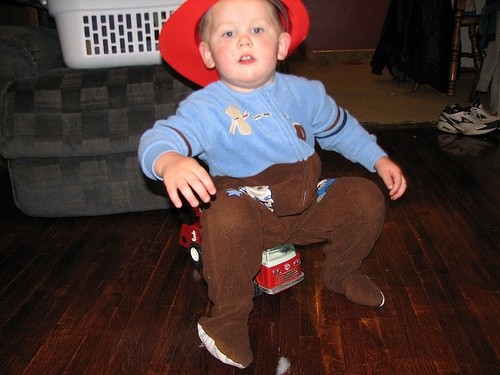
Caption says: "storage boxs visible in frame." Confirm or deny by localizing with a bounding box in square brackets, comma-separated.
[41, 0, 188, 70]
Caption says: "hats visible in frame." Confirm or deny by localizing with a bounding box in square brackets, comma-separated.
[159, 0, 310, 85]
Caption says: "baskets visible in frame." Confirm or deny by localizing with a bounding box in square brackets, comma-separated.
[44, 0, 187, 69]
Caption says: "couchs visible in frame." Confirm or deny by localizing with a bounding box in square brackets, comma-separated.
[0, 25, 203, 218]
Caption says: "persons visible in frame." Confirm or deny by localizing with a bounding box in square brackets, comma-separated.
[138, 0, 407, 368]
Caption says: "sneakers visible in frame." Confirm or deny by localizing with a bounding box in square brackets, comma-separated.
[437, 101, 500, 137]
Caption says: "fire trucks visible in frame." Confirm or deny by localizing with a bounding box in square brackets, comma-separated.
[178, 206, 305, 297]
